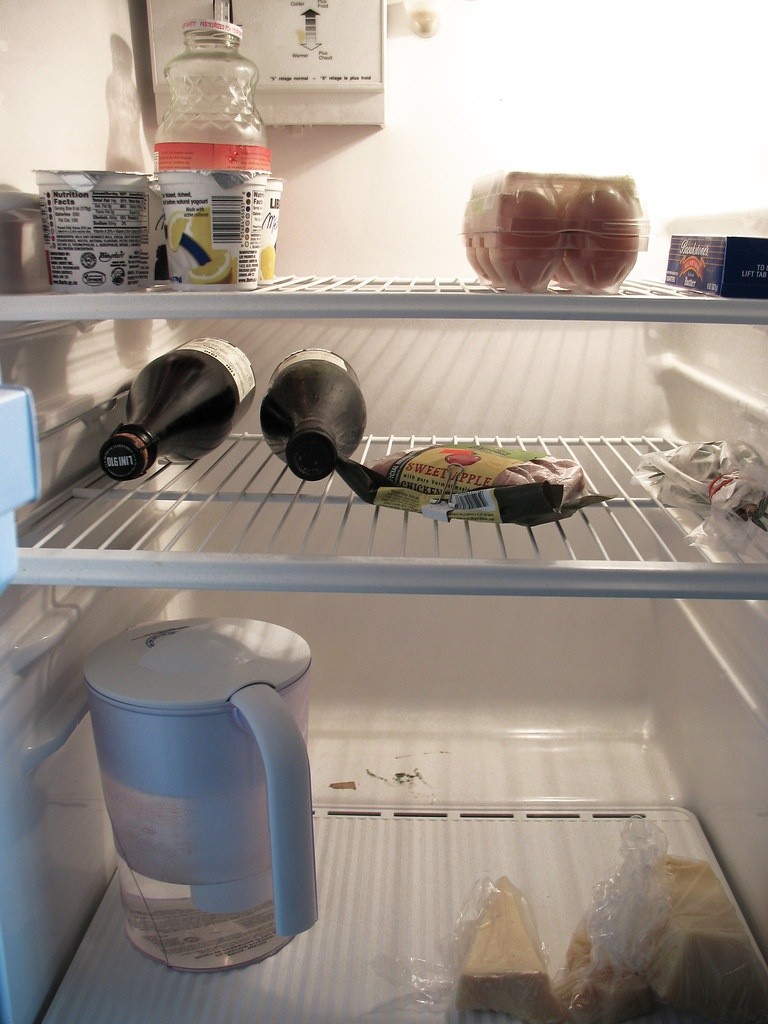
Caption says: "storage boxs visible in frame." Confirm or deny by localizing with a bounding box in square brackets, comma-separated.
[665, 235, 768, 297]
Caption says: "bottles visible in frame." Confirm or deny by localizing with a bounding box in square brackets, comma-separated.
[98, 336, 256, 483]
[151, 15, 272, 188]
[259, 348, 367, 482]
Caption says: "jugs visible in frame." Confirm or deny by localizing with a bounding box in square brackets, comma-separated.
[81, 615, 319, 973]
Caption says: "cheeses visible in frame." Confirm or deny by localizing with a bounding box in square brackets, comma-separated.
[456, 859, 764, 1024]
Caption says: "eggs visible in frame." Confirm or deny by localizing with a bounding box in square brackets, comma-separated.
[463, 174, 639, 289]
